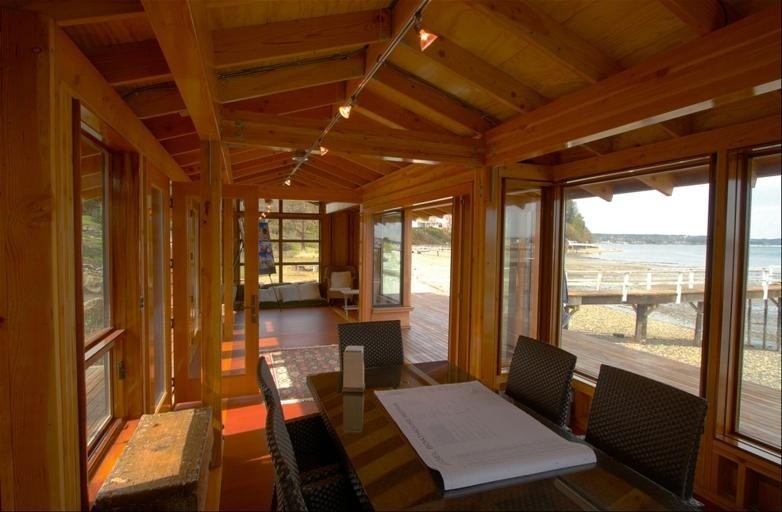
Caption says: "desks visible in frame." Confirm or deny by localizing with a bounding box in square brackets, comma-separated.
[305, 360, 706, 510]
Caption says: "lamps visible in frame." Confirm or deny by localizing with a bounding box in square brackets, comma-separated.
[412, 11, 440, 54]
[317, 94, 356, 157]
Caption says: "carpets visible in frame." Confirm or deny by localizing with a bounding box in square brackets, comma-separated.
[258, 343, 341, 406]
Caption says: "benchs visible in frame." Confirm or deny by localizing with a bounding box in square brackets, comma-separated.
[95, 406, 216, 511]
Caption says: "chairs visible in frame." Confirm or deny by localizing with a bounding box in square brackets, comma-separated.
[503, 334, 578, 427]
[585, 363, 709, 511]
[255, 355, 347, 477]
[323, 264, 357, 304]
[264, 406, 372, 511]
[336, 320, 404, 368]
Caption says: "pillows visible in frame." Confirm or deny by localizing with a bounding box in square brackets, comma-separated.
[258, 281, 321, 303]
